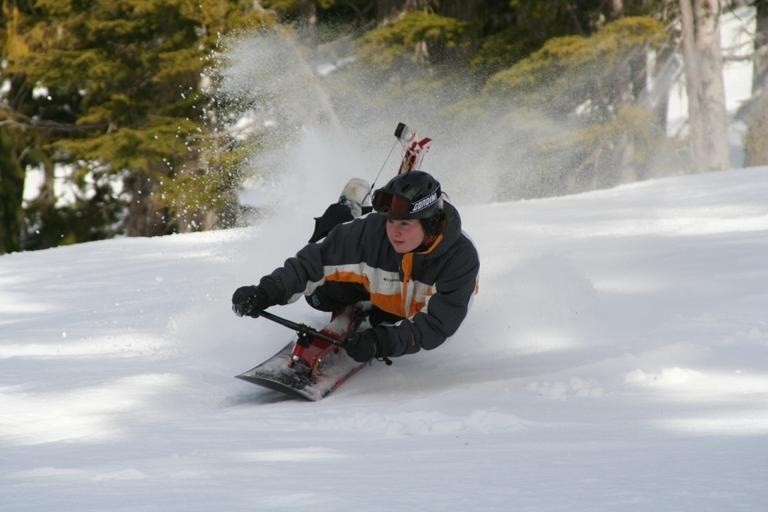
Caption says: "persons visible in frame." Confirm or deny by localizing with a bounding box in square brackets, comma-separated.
[231, 169, 480, 361]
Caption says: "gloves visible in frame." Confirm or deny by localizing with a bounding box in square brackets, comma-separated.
[232, 286, 268, 317]
[343, 328, 390, 362]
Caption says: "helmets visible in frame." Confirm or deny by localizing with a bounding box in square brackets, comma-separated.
[382, 172, 445, 221]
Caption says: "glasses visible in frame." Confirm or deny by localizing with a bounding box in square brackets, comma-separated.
[371, 185, 441, 215]
